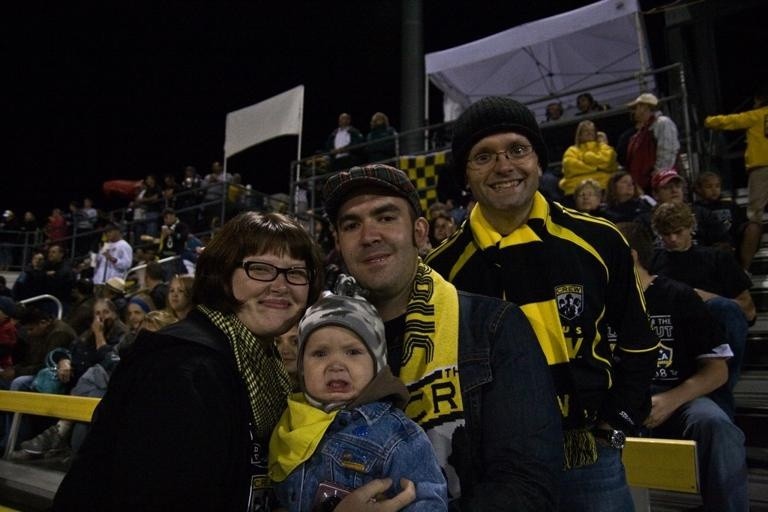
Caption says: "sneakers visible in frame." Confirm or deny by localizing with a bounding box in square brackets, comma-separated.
[21, 426, 70, 454]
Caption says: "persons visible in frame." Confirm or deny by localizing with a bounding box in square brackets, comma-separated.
[319, 167, 563, 512]
[597, 223, 750, 511]
[0, 163, 256, 470]
[53, 212, 416, 512]
[266, 290, 450, 512]
[326, 93, 768, 320]
[426, 95, 659, 511]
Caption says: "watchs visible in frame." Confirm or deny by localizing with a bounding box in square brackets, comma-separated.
[595, 429, 626, 449]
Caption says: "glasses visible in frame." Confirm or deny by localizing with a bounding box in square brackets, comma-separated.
[240, 261, 311, 285]
[467, 144, 536, 166]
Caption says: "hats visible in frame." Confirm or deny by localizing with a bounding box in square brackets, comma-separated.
[450, 95, 548, 185]
[296, 274, 388, 412]
[652, 169, 685, 186]
[625, 93, 658, 106]
[323, 164, 423, 216]
[106, 276, 125, 291]
